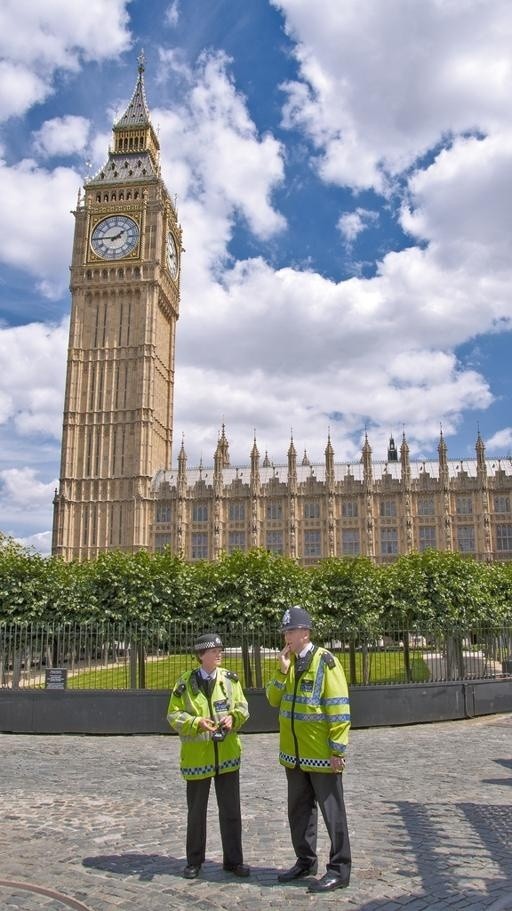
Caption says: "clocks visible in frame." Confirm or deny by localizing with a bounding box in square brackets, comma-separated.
[87, 211, 147, 264]
[164, 226, 181, 285]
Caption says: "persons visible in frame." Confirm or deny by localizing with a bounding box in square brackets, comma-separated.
[264, 607, 353, 893]
[166, 632, 251, 879]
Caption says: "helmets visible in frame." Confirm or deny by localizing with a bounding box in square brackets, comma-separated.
[277, 608, 312, 631]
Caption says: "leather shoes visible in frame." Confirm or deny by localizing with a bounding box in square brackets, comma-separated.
[278, 865, 317, 882]
[308, 876, 349, 892]
[223, 863, 249, 876]
[184, 863, 201, 878]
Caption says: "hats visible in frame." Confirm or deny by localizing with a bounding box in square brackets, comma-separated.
[194, 634, 223, 651]
